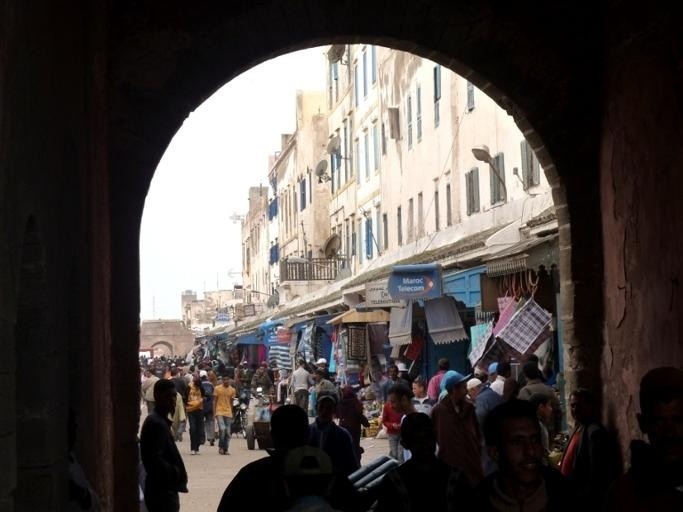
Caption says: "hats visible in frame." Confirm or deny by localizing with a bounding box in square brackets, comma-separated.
[440, 370, 472, 392]
[316, 358, 327, 365]
[466, 378, 481, 390]
[316, 391, 338, 404]
[200, 370, 209, 378]
[398, 362, 408, 371]
[488, 363, 498, 375]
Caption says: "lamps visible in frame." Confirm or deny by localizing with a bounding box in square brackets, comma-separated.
[469, 142, 506, 198]
[314, 160, 331, 185]
[512, 165, 532, 199]
[325, 134, 349, 161]
[322, 233, 348, 264]
[324, 43, 350, 68]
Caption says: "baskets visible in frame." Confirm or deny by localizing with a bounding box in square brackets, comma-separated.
[364, 426, 378, 436]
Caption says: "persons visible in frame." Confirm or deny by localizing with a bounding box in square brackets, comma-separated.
[215, 404, 369, 510]
[454, 400, 608, 511]
[139, 352, 370, 455]
[276, 488, 338, 510]
[559, 387, 625, 482]
[381, 352, 566, 456]
[375, 411, 452, 510]
[138, 378, 189, 511]
[623, 363, 683, 510]
[308, 395, 360, 472]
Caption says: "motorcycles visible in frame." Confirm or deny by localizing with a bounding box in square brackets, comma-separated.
[226, 392, 249, 442]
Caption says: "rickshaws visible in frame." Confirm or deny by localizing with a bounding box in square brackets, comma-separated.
[243, 394, 276, 452]
